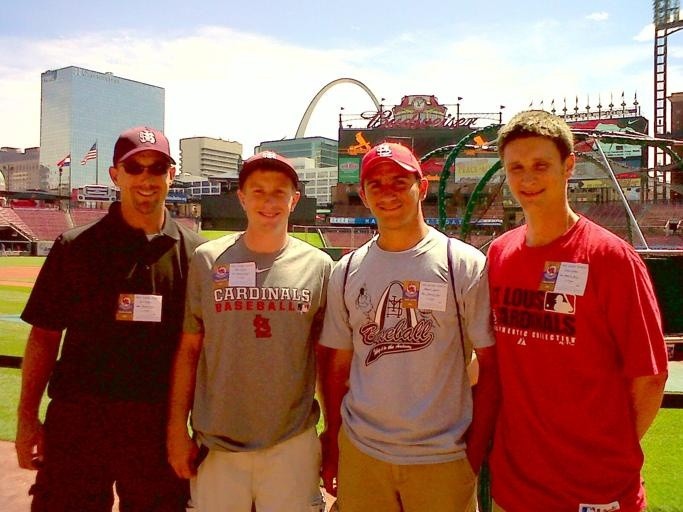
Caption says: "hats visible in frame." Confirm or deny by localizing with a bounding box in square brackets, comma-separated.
[241, 151, 299, 182]
[360, 143, 423, 180]
[113, 128, 176, 166]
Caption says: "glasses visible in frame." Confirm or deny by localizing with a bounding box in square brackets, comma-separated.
[122, 161, 169, 175]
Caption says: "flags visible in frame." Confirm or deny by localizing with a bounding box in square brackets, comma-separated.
[57, 155, 71, 166]
[81, 142, 96, 165]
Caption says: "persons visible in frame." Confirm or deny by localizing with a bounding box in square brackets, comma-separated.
[486, 110, 666, 512]
[323, 142, 495, 512]
[16, 126, 208, 512]
[167, 148, 331, 512]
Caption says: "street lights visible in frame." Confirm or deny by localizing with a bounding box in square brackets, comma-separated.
[58, 168, 62, 210]
[3, 165, 14, 192]
